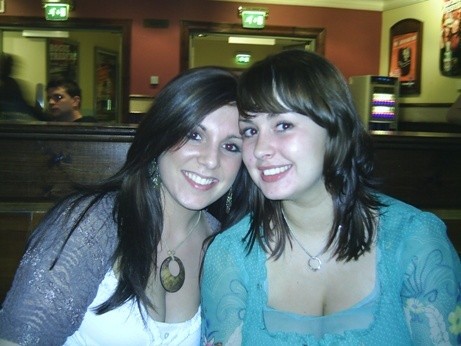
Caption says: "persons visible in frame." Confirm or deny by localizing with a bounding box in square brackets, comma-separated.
[0, 78, 99, 123]
[0, 65, 266, 346]
[199, 50, 461, 346]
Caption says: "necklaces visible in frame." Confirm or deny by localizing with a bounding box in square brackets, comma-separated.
[156, 210, 202, 292]
[279, 209, 346, 270]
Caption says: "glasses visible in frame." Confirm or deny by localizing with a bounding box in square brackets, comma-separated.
[46, 94, 63, 102]
